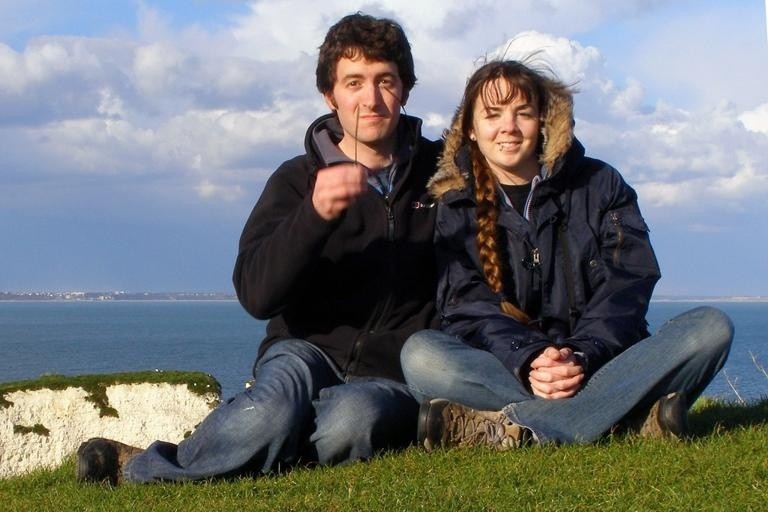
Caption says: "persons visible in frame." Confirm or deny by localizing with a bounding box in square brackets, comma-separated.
[79, 10, 439, 489]
[398, 41, 733, 453]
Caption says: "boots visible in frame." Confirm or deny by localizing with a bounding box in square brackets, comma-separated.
[77, 436, 146, 487]
[626, 389, 688, 440]
[419, 399, 530, 452]
